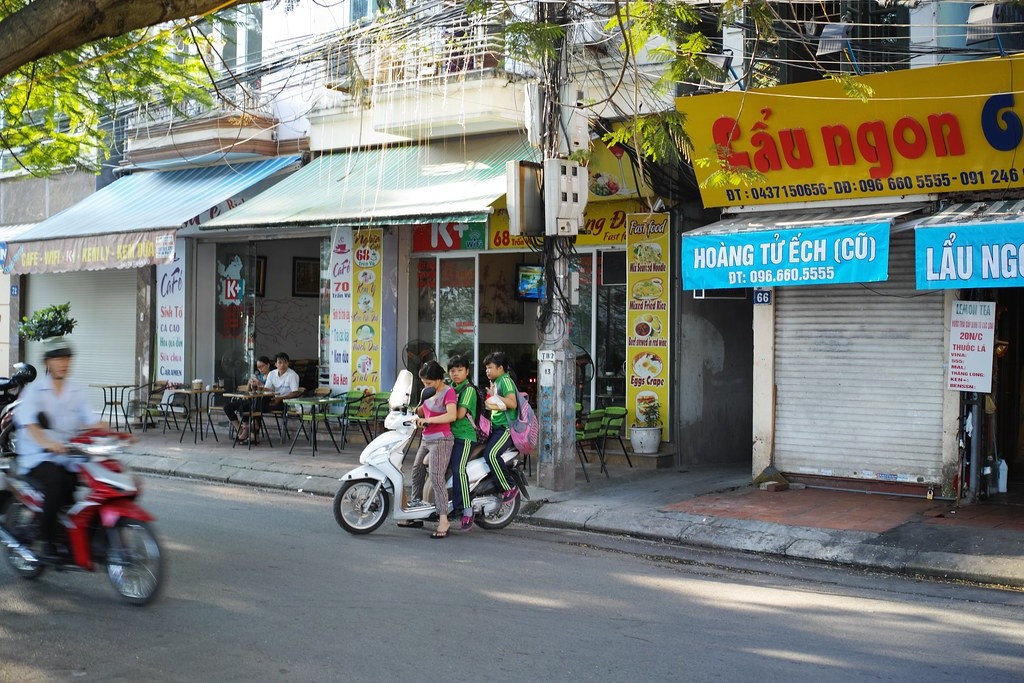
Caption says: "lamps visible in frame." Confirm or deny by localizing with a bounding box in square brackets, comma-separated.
[697, 49, 745, 91]
[654, 196, 666, 212]
[816, 14, 864, 77]
[965, 2, 1008, 58]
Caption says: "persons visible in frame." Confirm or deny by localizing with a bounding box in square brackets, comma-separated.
[12, 337, 136, 556]
[446, 355, 478, 531]
[252, 352, 299, 434]
[224, 356, 273, 442]
[483, 351, 520, 503]
[397, 360, 457, 539]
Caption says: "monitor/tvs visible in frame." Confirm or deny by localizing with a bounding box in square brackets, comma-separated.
[513, 262, 546, 301]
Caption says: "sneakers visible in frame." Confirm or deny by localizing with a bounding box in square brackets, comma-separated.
[462, 512, 475, 531]
[502, 485, 519, 504]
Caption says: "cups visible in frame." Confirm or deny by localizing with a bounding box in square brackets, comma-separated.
[335, 243, 346, 250]
[212, 382, 218, 390]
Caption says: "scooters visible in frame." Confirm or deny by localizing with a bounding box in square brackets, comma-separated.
[0, 362, 37, 453]
[333, 369, 531, 536]
[0, 427, 169, 607]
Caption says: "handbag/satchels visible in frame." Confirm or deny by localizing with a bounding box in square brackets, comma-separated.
[485, 383, 506, 411]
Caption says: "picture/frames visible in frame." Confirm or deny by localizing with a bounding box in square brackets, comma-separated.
[227, 254, 267, 297]
[292, 256, 320, 297]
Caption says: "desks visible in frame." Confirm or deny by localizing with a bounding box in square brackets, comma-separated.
[173, 388, 227, 444]
[88, 384, 139, 435]
[223, 392, 275, 450]
[597, 374, 624, 391]
[283, 397, 345, 456]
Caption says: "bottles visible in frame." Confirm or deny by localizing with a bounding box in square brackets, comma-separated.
[997, 459, 1008, 492]
[981, 455, 999, 494]
[252, 379, 257, 394]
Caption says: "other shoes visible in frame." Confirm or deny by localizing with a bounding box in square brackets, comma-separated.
[40, 543, 58, 556]
[237, 427, 260, 444]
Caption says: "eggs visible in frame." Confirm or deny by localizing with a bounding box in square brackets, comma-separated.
[641, 360, 650, 368]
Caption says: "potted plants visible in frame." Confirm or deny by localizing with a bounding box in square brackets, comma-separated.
[629, 402, 663, 453]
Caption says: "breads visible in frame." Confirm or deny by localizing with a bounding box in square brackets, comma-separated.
[644, 314, 660, 331]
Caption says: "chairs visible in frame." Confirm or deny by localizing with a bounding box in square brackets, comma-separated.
[124, 381, 225, 438]
[229, 385, 390, 450]
[576, 402, 633, 482]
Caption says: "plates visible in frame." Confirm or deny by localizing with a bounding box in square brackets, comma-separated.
[632, 242, 667, 424]
[355, 247, 381, 397]
[333, 248, 350, 254]
[589, 172, 621, 196]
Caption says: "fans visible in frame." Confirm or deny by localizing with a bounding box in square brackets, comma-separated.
[402, 339, 438, 405]
[573, 343, 595, 403]
[222, 347, 252, 392]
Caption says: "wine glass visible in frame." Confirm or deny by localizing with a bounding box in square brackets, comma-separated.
[602, 135, 637, 196]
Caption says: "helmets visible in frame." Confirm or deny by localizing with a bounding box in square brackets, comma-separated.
[43, 339, 73, 359]
[20, 363, 37, 382]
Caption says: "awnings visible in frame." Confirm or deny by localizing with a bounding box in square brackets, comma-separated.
[914, 199, 1024, 291]
[1, 156, 302, 275]
[681, 206, 926, 290]
[198, 133, 543, 231]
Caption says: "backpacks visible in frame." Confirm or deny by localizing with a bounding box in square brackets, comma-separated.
[497, 376, 538, 455]
[456, 383, 493, 445]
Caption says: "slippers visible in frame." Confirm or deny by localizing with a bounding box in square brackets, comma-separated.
[430, 529, 450, 539]
[398, 520, 423, 528]
